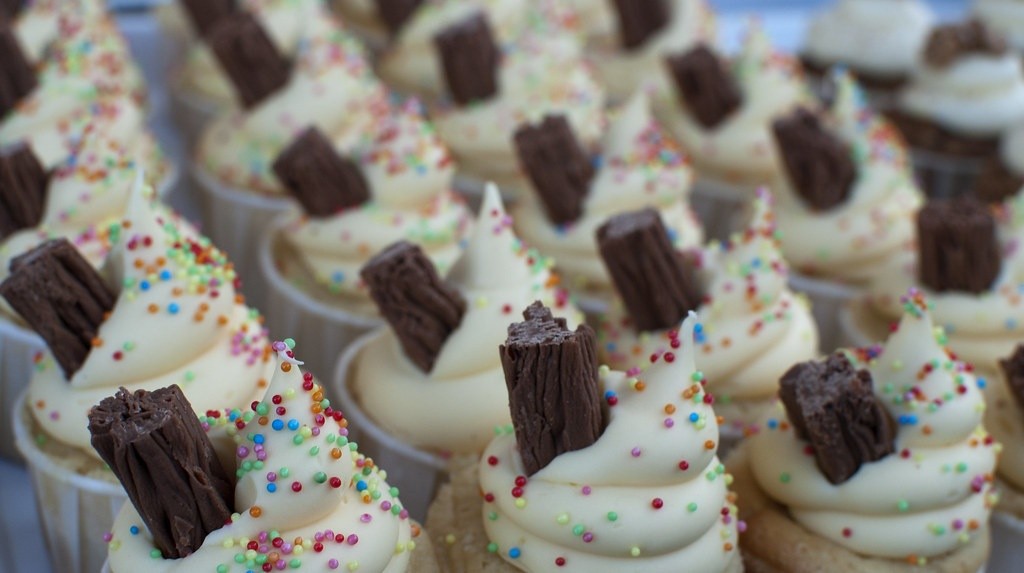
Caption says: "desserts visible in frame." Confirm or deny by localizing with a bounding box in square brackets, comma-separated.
[0, 0, 1024, 573]
